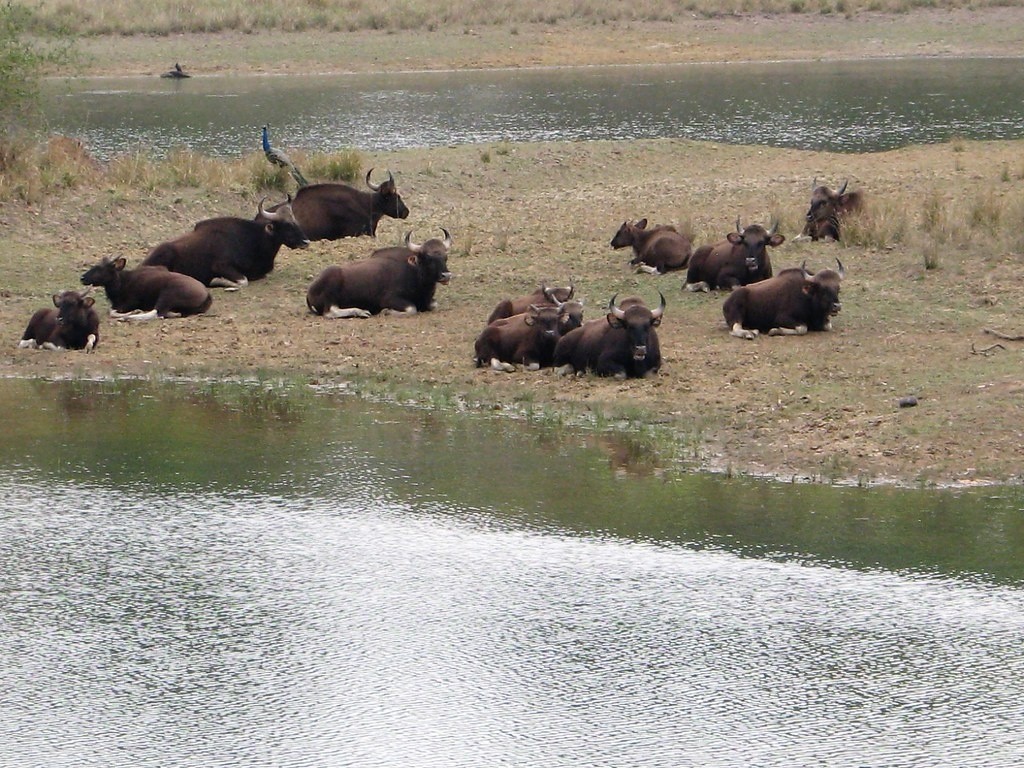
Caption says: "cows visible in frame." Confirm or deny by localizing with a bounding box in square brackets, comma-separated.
[611, 218, 692, 276]
[722, 258, 845, 340]
[679, 215, 785, 293]
[136, 192, 311, 289]
[17, 284, 100, 355]
[291, 168, 410, 241]
[306, 227, 452, 319]
[790, 177, 865, 244]
[80, 252, 213, 321]
[473, 277, 666, 380]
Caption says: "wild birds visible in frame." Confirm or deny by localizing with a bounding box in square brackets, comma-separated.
[258, 122, 307, 186]
